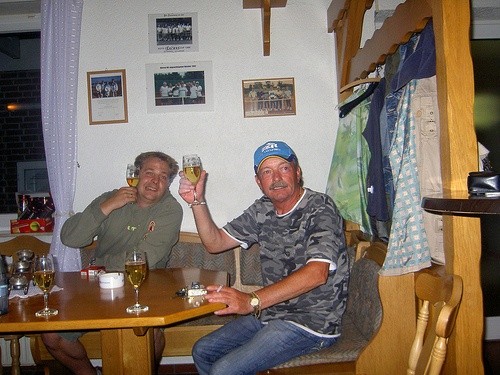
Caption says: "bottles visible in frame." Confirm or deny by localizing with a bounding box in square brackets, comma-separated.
[0, 256, 8, 315]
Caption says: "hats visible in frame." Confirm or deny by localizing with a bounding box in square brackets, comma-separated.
[253, 140, 296, 175]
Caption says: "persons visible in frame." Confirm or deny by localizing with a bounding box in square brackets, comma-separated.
[41, 151, 184, 375]
[157, 22, 192, 41]
[248, 84, 293, 113]
[95, 79, 119, 98]
[179, 139, 350, 375]
[159, 80, 203, 103]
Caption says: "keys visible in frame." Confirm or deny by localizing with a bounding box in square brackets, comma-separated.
[176, 280, 200, 296]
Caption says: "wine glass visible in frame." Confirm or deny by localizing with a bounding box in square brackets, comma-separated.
[183, 155, 206, 206]
[34, 257, 58, 316]
[126, 250, 148, 312]
[126, 164, 141, 205]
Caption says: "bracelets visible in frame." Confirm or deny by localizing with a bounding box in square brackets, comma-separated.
[188, 201, 207, 208]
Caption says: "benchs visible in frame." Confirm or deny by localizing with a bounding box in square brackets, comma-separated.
[0, 230, 390, 375]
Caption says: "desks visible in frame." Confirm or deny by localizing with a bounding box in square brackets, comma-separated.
[0, 269, 230, 375]
[421, 190, 500, 215]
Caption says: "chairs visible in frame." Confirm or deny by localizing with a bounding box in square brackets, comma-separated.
[406, 272, 464, 375]
[0, 234, 52, 375]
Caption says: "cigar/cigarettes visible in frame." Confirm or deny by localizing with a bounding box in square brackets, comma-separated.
[216, 284, 223, 292]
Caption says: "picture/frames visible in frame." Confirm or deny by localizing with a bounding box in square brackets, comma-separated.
[87, 69, 128, 125]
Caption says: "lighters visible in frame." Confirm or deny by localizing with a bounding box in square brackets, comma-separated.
[187, 288, 207, 296]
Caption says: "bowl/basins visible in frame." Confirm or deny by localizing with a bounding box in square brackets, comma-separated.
[99, 273, 124, 288]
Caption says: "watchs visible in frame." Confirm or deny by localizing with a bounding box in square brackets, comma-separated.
[249, 291, 261, 318]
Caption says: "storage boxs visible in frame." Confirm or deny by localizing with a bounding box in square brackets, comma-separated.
[10, 217, 54, 234]
